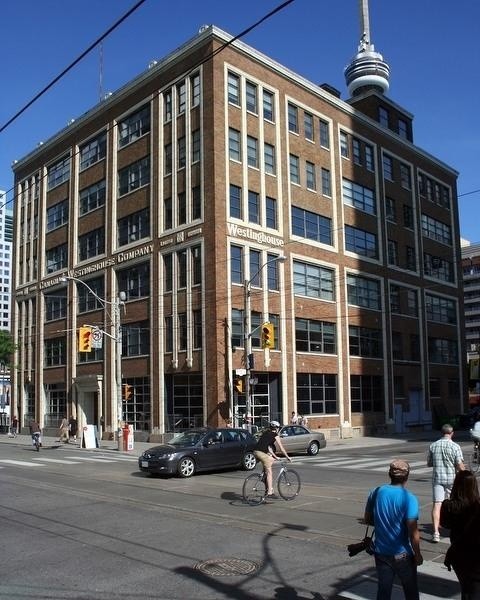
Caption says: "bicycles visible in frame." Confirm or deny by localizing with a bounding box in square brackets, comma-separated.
[240, 458, 302, 507]
[468, 439, 480, 474]
[32, 434, 41, 451]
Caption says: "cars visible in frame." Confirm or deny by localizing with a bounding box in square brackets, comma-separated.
[137, 427, 258, 479]
[254, 423, 327, 456]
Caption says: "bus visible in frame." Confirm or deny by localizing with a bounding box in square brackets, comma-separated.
[468, 378, 480, 406]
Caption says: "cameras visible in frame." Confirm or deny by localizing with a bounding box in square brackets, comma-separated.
[348, 537, 374, 557]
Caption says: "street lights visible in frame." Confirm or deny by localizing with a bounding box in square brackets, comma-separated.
[244, 255, 289, 423]
[56, 274, 122, 442]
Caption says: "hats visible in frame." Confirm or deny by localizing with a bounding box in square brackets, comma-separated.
[391, 460, 408, 476]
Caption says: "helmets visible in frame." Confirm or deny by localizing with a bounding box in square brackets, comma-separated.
[270, 421, 280, 427]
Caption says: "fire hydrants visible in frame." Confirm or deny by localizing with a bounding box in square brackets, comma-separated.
[122, 424, 130, 452]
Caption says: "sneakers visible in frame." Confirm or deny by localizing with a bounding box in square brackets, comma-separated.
[267, 494, 277, 498]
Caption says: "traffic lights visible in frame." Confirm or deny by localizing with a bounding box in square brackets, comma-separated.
[125, 385, 134, 400]
[261, 324, 275, 349]
[79, 327, 91, 351]
[234, 378, 243, 395]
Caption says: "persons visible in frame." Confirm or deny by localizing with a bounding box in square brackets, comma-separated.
[11, 415, 19, 438]
[438, 469, 480, 600]
[283, 429, 288, 434]
[29, 418, 42, 449]
[426, 423, 466, 544]
[469, 419, 480, 464]
[208, 434, 216, 444]
[252, 419, 293, 499]
[289, 411, 298, 431]
[363, 457, 423, 600]
[68, 415, 78, 443]
[57, 415, 71, 444]
[298, 413, 309, 433]
